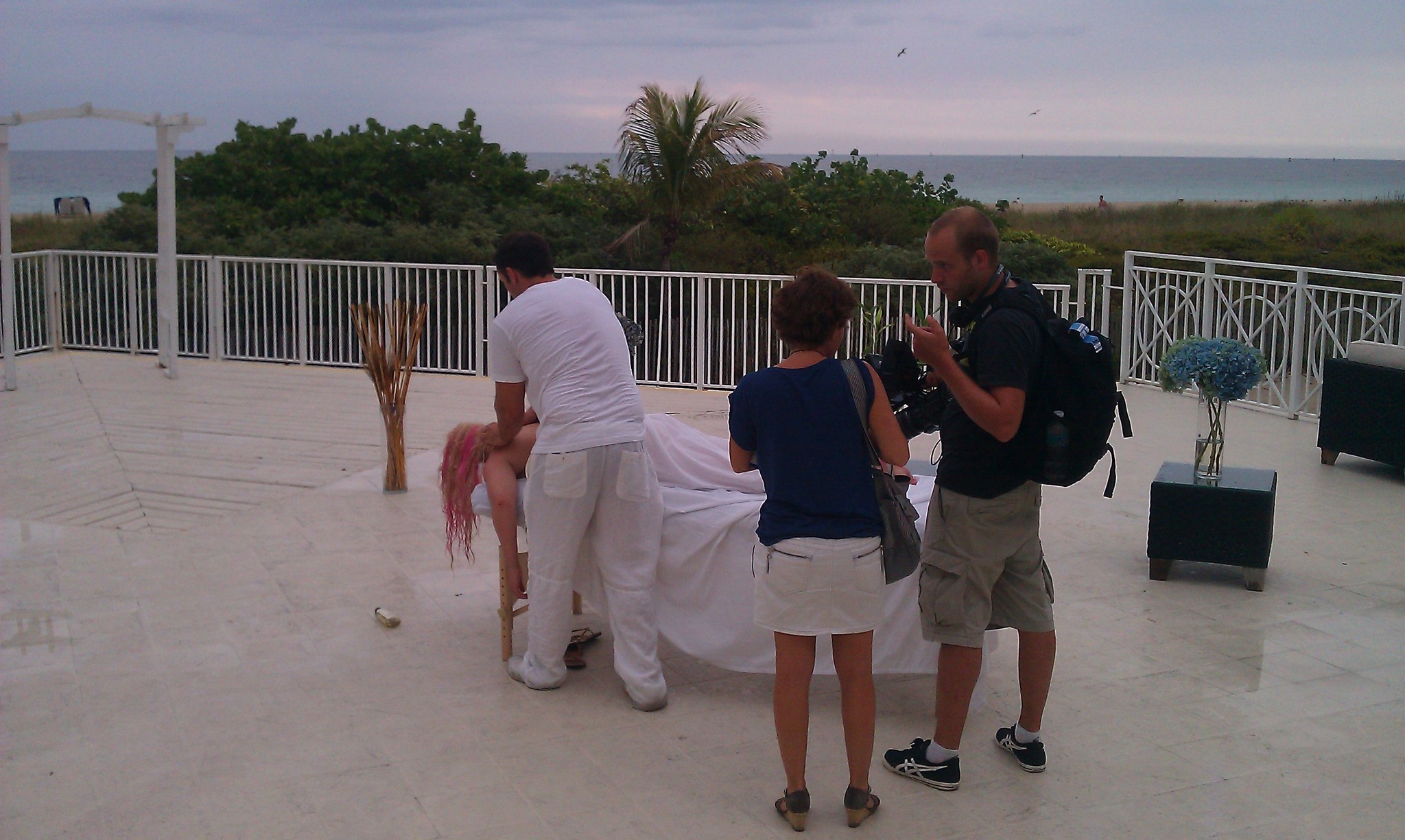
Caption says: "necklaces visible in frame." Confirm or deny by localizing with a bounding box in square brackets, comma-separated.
[788, 348, 826, 360]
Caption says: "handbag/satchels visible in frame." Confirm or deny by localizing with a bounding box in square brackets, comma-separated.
[872, 465, 921, 586]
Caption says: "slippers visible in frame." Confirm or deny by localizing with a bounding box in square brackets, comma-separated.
[563, 626, 602, 668]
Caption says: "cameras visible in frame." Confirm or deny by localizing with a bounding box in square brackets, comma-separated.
[891, 379, 946, 441]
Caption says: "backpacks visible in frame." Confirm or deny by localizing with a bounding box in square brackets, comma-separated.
[995, 271, 1134, 500]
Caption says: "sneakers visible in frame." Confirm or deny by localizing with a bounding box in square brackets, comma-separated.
[507, 654, 560, 690]
[883, 736, 962, 791]
[994, 723, 1048, 772]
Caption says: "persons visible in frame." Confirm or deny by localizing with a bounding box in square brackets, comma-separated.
[874, 203, 1068, 795]
[484, 226, 674, 713]
[1098, 195, 1110, 210]
[1017, 197, 1020, 204]
[440, 405, 765, 597]
[726, 262, 912, 834]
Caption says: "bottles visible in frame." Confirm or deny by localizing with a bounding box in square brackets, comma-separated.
[374, 605, 402, 627]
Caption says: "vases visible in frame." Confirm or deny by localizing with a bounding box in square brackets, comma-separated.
[351, 296, 428, 494]
[1156, 336, 1268, 482]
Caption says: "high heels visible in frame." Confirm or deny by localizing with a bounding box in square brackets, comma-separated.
[774, 784, 811, 832]
[842, 783, 881, 828]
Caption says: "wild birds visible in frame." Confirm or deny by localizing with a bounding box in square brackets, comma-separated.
[1288, 198, 1353, 205]
[1214, 199, 1217, 202]
[1239, 200, 1253, 202]
[898, 47, 907, 58]
[1029, 109, 1041, 117]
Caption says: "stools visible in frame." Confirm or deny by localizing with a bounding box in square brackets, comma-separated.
[1146, 460, 1278, 594]
[1315, 338, 1405, 471]
